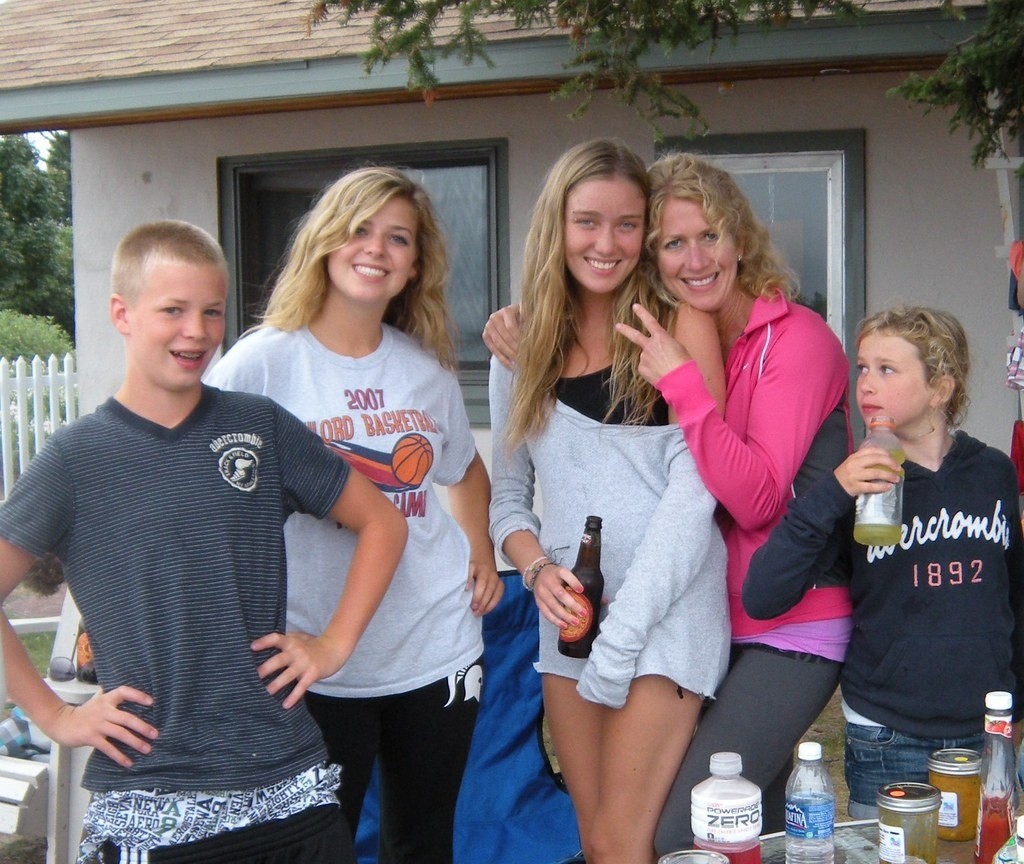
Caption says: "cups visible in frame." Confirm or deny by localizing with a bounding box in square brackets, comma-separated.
[659, 848, 729, 864]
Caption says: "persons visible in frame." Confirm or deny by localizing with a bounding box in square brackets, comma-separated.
[740, 303, 1024, 825]
[201, 167, 506, 864]
[1, 221, 409, 864]
[640, 151, 855, 863]
[489, 134, 732, 864]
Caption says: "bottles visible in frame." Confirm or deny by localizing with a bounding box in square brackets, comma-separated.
[556, 514, 607, 660]
[973, 689, 1017, 864]
[852, 417, 906, 546]
[688, 751, 763, 864]
[926, 746, 983, 843]
[784, 741, 836, 864]
[875, 781, 940, 864]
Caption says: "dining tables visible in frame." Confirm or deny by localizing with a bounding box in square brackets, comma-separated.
[757, 814, 1024, 864]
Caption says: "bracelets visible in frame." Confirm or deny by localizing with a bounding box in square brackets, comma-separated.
[522, 545, 569, 592]
